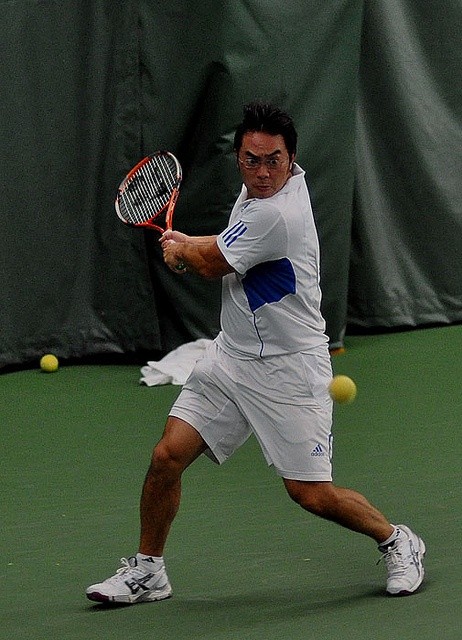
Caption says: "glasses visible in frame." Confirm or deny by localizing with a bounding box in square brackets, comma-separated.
[238, 156, 288, 169]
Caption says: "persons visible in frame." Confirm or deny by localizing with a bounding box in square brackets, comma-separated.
[84, 105, 428, 606]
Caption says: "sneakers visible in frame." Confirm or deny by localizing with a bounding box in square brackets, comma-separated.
[376, 523, 426, 597]
[85, 556, 173, 605]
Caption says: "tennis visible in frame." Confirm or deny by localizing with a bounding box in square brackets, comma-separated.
[40, 353, 59, 373]
[328, 375, 357, 404]
